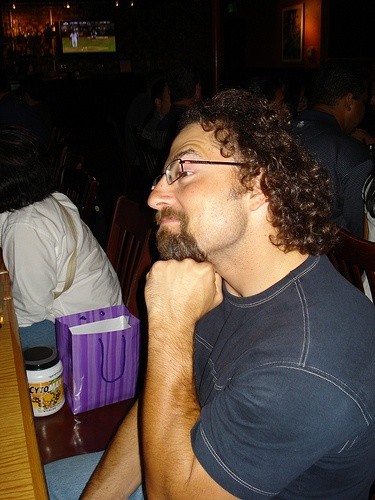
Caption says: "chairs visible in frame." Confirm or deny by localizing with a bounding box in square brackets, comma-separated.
[31, 135, 159, 462]
[328, 222, 375, 304]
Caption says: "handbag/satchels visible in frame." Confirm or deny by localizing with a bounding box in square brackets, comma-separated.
[54, 304, 140, 413]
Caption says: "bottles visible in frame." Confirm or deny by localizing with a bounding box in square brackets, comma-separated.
[22, 344, 66, 418]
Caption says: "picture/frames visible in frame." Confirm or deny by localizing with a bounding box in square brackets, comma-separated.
[279, 1, 304, 66]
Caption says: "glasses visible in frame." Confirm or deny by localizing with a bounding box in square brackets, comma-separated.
[154, 157, 245, 187]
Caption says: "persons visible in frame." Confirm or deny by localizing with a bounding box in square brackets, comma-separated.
[1, 57, 375, 352]
[44, 87, 375, 500]
[70, 29, 97, 47]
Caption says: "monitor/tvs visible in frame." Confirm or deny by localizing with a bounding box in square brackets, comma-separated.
[58, 18, 118, 55]
